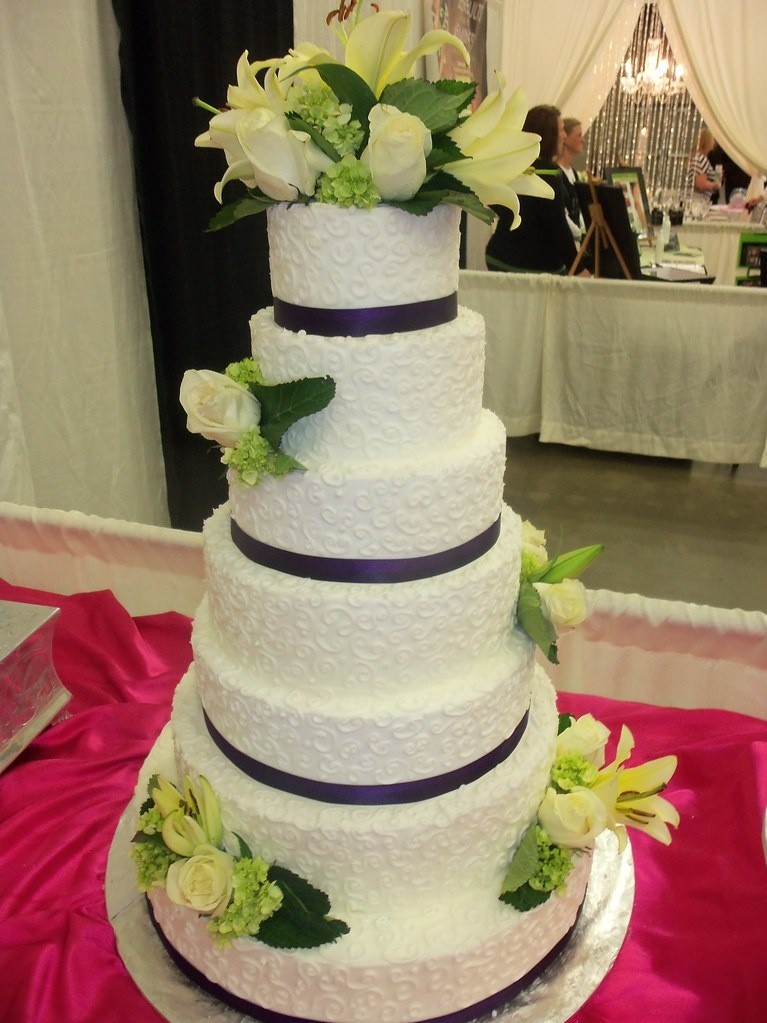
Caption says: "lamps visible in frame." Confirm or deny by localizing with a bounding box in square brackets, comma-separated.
[617, 37, 687, 98]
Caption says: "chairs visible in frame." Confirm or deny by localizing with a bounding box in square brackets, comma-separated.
[751, 200, 767, 230]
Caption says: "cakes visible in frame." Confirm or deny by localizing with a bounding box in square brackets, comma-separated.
[123, 0, 682, 1023]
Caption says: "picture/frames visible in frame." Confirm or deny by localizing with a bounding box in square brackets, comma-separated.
[604, 167, 655, 241]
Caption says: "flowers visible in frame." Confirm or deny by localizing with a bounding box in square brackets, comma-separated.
[127, 768, 349, 953]
[493, 708, 682, 914]
[174, 356, 337, 490]
[190, 0, 556, 240]
[508, 512, 606, 670]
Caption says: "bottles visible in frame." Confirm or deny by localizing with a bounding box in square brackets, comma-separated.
[662, 215, 671, 245]
[649, 202, 684, 225]
[654, 230, 665, 260]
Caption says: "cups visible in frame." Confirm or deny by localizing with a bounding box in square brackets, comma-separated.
[646, 189, 678, 208]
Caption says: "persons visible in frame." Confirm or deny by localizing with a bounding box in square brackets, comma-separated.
[603, 128, 629, 167]
[688, 127, 752, 206]
[630, 182, 646, 231]
[483, 105, 590, 278]
[553, 118, 585, 256]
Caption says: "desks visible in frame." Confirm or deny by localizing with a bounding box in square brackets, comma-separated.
[682, 221, 766, 288]
[634, 238, 707, 267]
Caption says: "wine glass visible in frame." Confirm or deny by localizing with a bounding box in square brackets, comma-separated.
[679, 188, 712, 221]
